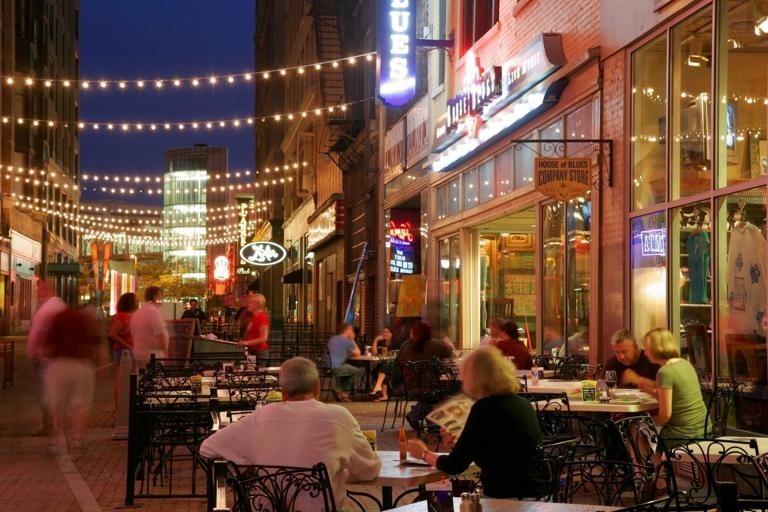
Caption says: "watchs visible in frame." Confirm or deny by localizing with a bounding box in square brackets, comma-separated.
[422, 451, 428, 461]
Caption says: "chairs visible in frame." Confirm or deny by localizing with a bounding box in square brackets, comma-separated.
[116, 289, 766, 512]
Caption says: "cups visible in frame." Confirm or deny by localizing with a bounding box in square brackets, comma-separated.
[383, 347, 388, 356]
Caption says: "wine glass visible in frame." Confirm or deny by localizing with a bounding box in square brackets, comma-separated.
[606, 370, 617, 402]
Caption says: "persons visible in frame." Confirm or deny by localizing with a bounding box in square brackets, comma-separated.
[181, 299, 208, 361]
[234, 287, 269, 368]
[319, 316, 534, 431]
[407, 347, 554, 502]
[629, 328, 713, 465]
[199, 356, 382, 512]
[557, 318, 589, 367]
[26, 287, 103, 454]
[107, 287, 170, 427]
[598, 329, 661, 474]
[748, 312, 768, 366]
[224, 287, 234, 323]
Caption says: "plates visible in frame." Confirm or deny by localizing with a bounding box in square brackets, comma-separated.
[612, 388, 642, 392]
[610, 397, 650, 404]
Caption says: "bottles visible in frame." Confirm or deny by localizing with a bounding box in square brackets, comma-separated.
[472, 492, 482, 512]
[537, 367, 544, 380]
[377, 345, 383, 355]
[400, 426, 407, 461]
[460, 492, 472, 512]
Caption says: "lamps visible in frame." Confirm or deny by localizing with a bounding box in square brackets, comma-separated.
[682, 32, 709, 68]
[750, 0, 767, 38]
